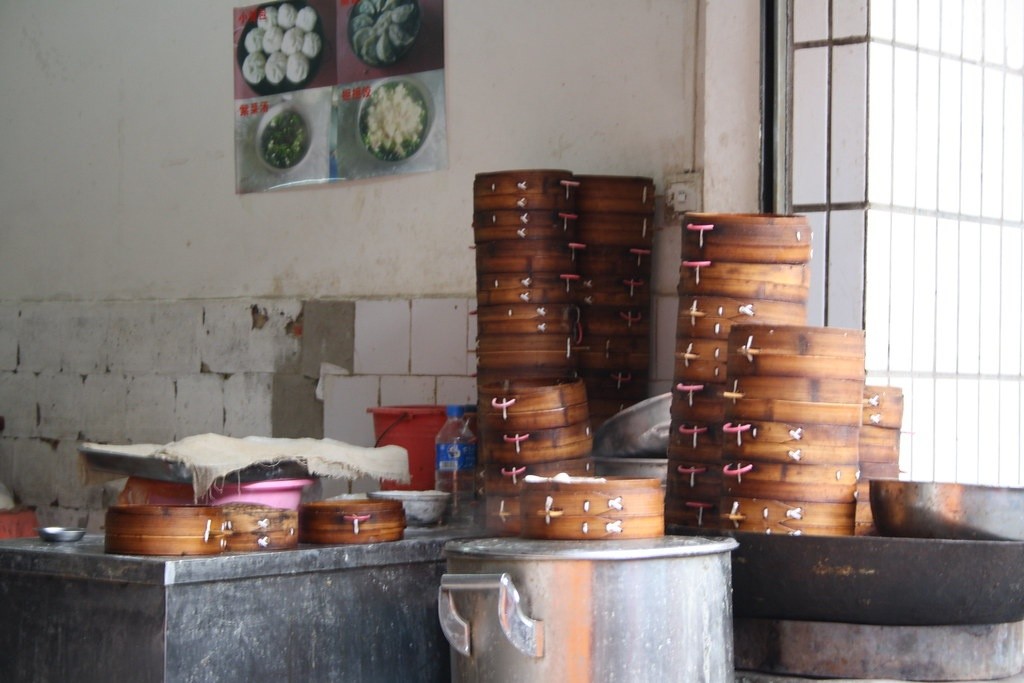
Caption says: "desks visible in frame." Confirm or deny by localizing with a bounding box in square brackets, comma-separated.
[0, 529, 496, 683]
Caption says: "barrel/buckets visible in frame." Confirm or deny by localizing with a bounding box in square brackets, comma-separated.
[367, 404, 448, 492]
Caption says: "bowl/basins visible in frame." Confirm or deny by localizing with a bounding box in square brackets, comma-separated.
[367, 490, 450, 527]
[356, 77, 436, 166]
[111, 478, 315, 510]
[255, 101, 317, 174]
[869, 479, 1024, 543]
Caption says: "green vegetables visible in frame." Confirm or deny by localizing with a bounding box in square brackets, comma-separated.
[258, 111, 307, 168]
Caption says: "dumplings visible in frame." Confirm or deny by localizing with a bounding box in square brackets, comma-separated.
[350, 0, 416, 64]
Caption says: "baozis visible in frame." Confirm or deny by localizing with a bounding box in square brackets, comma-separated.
[241, 3, 322, 84]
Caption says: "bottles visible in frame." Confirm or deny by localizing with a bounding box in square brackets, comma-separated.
[435, 404, 475, 532]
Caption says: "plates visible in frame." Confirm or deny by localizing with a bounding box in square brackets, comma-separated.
[36, 527, 87, 542]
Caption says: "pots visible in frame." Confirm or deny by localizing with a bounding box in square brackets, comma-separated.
[437, 536, 739, 683]
